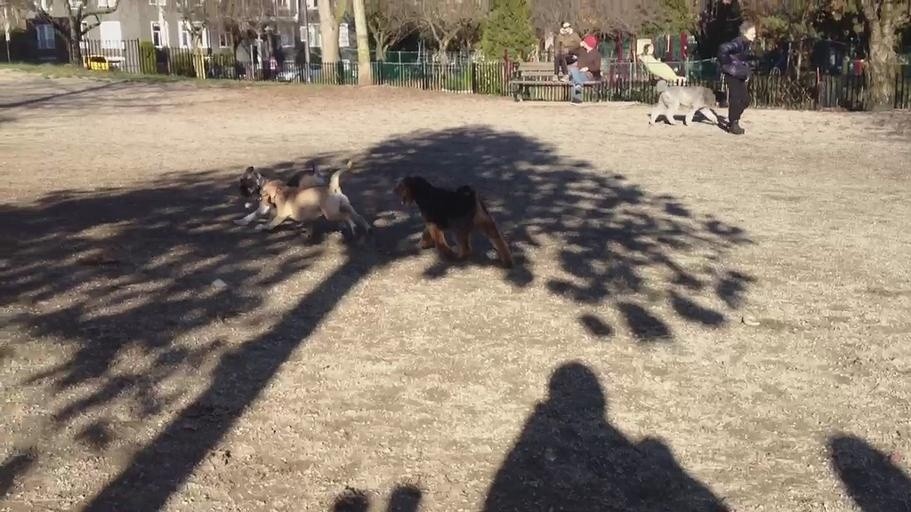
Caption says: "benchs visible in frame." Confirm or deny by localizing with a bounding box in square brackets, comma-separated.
[509, 81, 601, 103]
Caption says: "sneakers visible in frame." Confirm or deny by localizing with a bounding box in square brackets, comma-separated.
[573, 85, 582, 102]
[552, 74, 569, 82]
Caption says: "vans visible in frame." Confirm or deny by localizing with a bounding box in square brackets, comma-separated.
[85, 55, 109, 72]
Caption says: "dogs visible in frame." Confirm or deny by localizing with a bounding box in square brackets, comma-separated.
[231, 159, 324, 228]
[393, 177, 514, 268]
[258, 160, 372, 241]
[649, 80, 719, 126]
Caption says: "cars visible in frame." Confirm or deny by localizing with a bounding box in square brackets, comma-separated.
[382, 64, 422, 78]
[609, 52, 683, 82]
[758, 38, 851, 74]
[273, 63, 343, 86]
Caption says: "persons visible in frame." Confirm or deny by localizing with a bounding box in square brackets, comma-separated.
[718, 19, 756, 134]
[567, 35, 601, 103]
[550, 21, 581, 82]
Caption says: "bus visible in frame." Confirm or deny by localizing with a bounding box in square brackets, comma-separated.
[344, 47, 431, 78]
[78, 54, 125, 70]
[267, 59, 352, 80]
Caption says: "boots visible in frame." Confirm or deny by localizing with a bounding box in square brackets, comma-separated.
[728, 120, 744, 134]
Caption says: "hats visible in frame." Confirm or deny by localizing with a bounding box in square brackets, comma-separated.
[583, 36, 596, 48]
